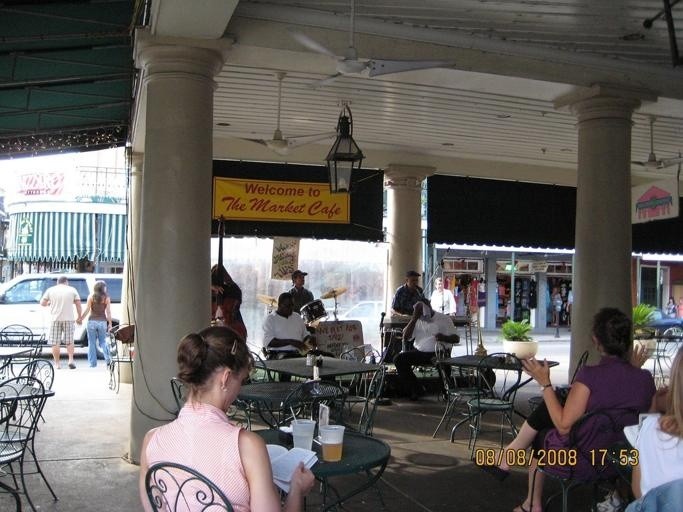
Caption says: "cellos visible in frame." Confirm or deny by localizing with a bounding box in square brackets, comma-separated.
[212, 215, 247, 343]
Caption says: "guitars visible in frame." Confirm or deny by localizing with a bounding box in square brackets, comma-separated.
[474, 320, 495, 389]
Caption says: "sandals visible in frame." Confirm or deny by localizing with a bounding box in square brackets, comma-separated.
[473, 456, 509, 483]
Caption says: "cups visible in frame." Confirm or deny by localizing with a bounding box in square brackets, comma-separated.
[320, 423, 345, 461]
[290, 419, 316, 451]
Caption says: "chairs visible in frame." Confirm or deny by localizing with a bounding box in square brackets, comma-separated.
[0, 322, 60, 512]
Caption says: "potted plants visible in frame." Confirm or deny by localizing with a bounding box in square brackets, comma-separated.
[632, 304, 661, 355]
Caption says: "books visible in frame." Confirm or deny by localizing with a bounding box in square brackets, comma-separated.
[265, 444, 319, 495]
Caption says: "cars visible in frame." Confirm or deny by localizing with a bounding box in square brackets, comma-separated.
[635, 307, 682, 341]
[323, 299, 387, 338]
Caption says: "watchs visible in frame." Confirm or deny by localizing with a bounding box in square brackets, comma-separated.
[537, 385, 552, 392]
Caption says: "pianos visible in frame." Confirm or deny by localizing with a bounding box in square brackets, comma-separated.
[382, 316, 472, 330]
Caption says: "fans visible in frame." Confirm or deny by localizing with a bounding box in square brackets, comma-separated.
[287, 0, 459, 94]
[632, 114, 683, 175]
[207, 66, 342, 155]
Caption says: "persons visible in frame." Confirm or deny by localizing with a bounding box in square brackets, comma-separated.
[76, 280, 112, 367]
[629, 345, 683, 498]
[211, 264, 247, 342]
[665, 297, 675, 320]
[287, 269, 313, 314]
[550, 286, 562, 326]
[392, 299, 460, 401]
[138, 325, 316, 512]
[428, 277, 456, 316]
[390, 271, 425, 319]
[566, 290, 573, 322]
[261, 292, 335, 382]
[675, 297, 682, 319]
[470, 306, 657, 512]
[39, 276, 82, 369]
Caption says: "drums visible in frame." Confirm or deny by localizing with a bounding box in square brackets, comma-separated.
[299, 299, 328, 337]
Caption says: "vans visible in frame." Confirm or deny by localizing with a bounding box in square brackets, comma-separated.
[0, 272, 127, 345]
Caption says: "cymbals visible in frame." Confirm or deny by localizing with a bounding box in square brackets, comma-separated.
[255, 293, 279, 307]
[320, 287, 347, 300]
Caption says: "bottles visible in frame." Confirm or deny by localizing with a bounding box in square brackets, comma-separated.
[504, 353, 517, 365]
[655, 328, 658, 336]
[305, 352, 321, 366]
[370, 353, 375, 364]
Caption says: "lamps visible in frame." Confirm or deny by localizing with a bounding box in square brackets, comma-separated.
[325, 98, 364, 194]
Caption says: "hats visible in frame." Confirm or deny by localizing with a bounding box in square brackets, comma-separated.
[292, 270, 308, 277]
[406, 271, 422, 277]
